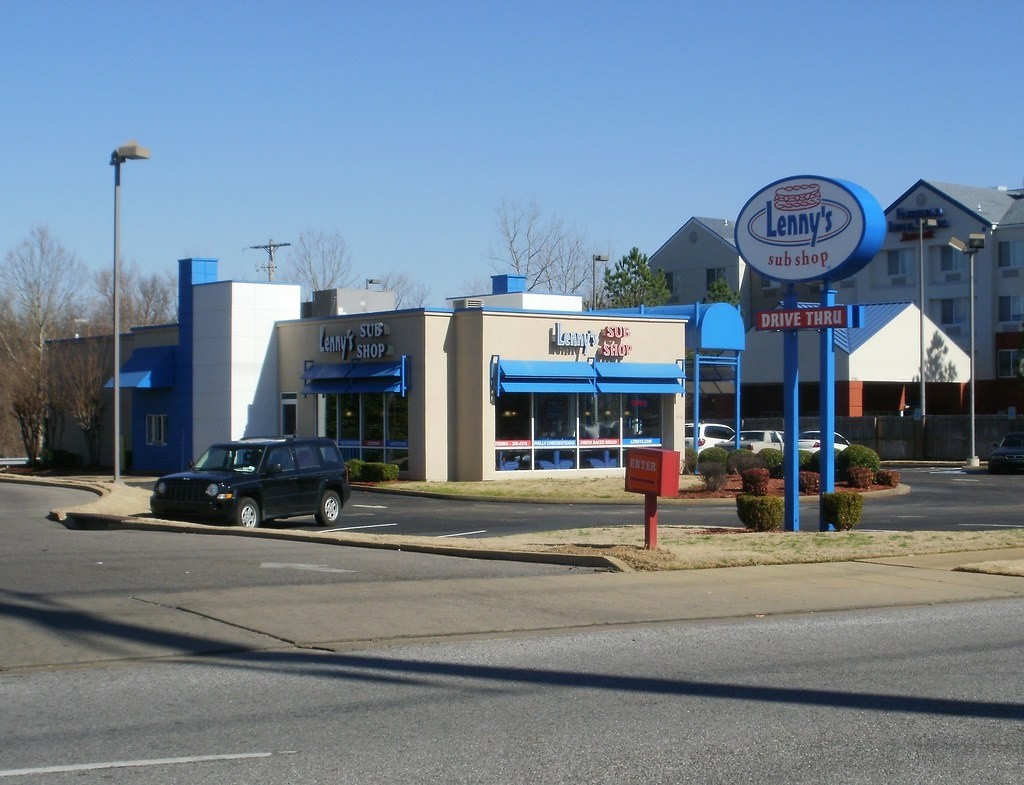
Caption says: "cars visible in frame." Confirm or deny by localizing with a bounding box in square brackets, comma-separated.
[714, 429, 785, 453]
[798, 431, 850, 452]
[989, 432, 1024, 473]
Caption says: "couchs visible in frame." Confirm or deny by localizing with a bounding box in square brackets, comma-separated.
[534, 450, 573, 469]
[583, 449, 617, 467]
[499, 451, 519, 470]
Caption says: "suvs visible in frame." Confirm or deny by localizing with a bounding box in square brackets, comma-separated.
[150, 437, 351, 530]
[684, 423, 743, 455]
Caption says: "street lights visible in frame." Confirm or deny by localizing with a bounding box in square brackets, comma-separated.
[592, 255, 609, 310]
[112, 144, 149, 484]
[949, 234, 986, 466]
[916, 217, 938, 458]
